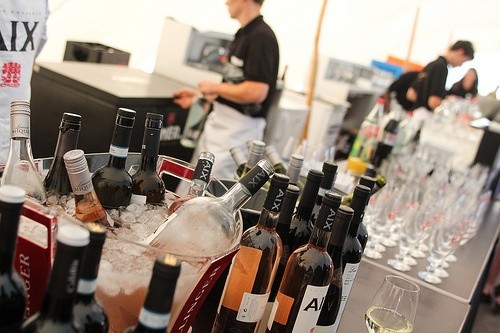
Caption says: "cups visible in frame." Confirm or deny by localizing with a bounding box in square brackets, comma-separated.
[364, 275, 419, 333]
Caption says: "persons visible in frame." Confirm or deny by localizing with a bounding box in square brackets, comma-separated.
[0, 0, 51, 165]
[173, 0, 280, 198]
[373, 41, 479, 167]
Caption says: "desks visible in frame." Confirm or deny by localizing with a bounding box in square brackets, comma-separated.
[335, 196, 500, 333]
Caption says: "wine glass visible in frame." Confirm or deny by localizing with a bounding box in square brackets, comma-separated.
[363, 140, 492, 285]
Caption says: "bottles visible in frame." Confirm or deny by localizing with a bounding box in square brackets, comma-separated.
[0, 100, 402, 333]
[217, 53, 261, 116]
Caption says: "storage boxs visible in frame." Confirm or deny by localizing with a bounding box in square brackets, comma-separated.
[30, 61, 194, 160]
[64, 41, 130, 65]
[369, 60, 404, 87]
